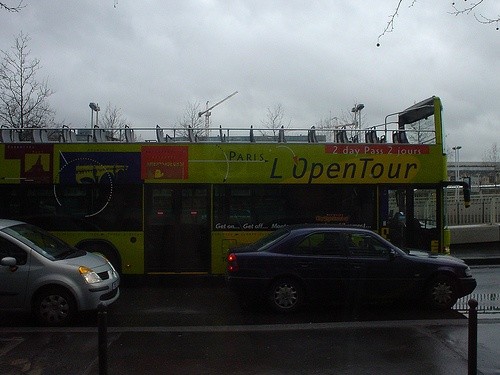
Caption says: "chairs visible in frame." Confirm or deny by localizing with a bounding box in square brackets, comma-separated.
[0, 124, 435, 142]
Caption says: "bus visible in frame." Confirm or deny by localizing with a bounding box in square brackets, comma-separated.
[0, 95, 471, 316]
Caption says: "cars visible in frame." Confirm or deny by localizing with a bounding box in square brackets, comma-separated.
[0, 219, 120, 330]
[226, 225, 477, 309]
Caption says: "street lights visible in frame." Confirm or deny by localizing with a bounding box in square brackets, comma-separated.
[452, 146, 462, 202]
[89, 100, 100, 127]
[351, 103, 364, 143]
